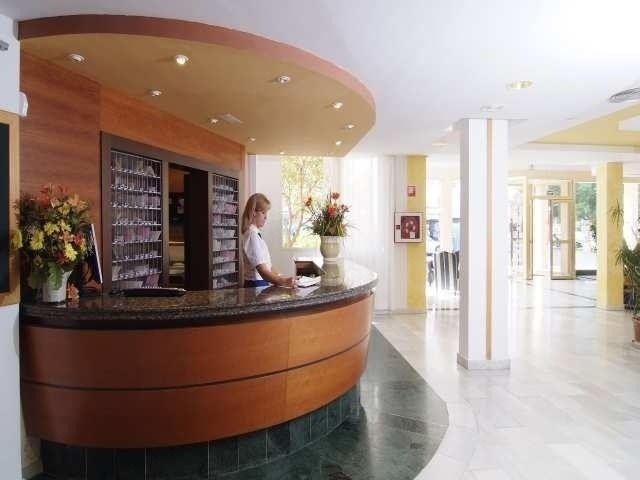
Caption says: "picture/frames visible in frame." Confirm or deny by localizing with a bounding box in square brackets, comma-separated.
[393, 212, 423, 243]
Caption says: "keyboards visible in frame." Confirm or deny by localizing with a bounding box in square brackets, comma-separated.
[125, 286, 185, 297]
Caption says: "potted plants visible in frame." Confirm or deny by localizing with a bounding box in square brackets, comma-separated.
[608, 197, 640, 345]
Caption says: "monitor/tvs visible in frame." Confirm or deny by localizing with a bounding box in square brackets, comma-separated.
[90, 224, 104, 284]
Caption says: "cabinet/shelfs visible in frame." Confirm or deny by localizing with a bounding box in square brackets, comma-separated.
[184, 171, 240, 292]
[101, 130, 171, 294]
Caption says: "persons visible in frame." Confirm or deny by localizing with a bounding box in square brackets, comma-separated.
[241, 193, 298, 288]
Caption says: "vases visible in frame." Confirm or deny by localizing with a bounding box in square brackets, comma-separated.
[320, 236, 342, 261]
[36, 262, 72, 304]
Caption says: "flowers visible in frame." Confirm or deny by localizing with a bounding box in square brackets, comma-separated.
[6, 180, 97, 300]
[298, 190, 360, 245]
